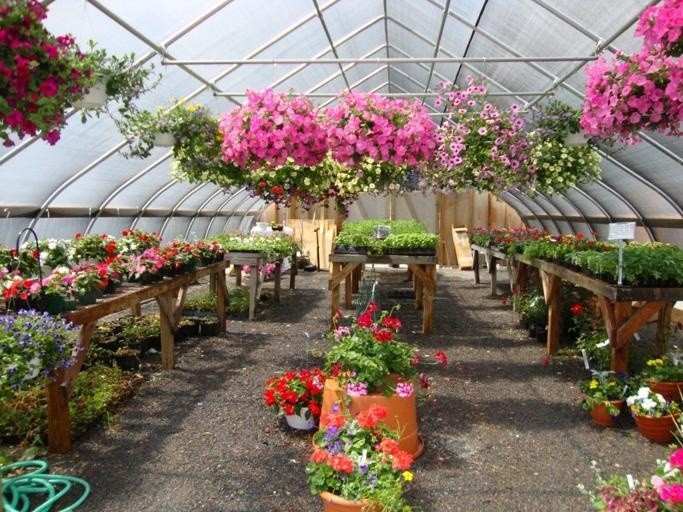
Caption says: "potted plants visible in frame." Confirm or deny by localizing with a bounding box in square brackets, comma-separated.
[332, 221, 441, 257]
[221, 233, 300, 260]
[0, 375, 142, 451]
[80, 287, 270, 375]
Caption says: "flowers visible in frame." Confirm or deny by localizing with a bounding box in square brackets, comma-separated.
[0, 2, 90, 152]
[303, 398, 417, 511]
[508, 283, 683, 421]
[261, 366, 328, 420]
[312, 302, 447, 398]
[0, 230, 229, 324]
[0, 308, 81, 392]
[69, 41, 164, 161]
[577, 0, 683, 148]
[470, 224, 682, 287]
[158, 77, 601, 217]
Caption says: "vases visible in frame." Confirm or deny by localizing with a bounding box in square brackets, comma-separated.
[5, 356, 42, 381]
[70, 69, 111, 111]
[309, 373, 427, 463]
[283, 405, 316, 431]
[589, 399, 624, 427]
[319, 491, 380, 512]
[630, 405, 681, 442]
